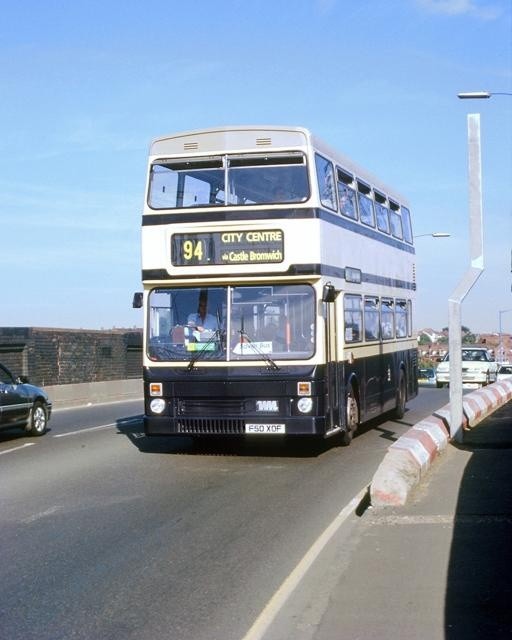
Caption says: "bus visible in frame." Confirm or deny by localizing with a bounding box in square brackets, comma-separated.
[131, 126, 419, 447]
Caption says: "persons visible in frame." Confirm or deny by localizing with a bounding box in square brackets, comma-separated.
[272, 186, 287, 201]
[186, 297, 225, 337]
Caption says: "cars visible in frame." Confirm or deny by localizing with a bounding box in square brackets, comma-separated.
[436, 348, 498, 387]
[0, 363, 51, 436]
[417, 368, 444, 388]
[497, 364, 512, 382]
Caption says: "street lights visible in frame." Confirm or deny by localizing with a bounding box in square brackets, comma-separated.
[414, 232, 451, 238]
